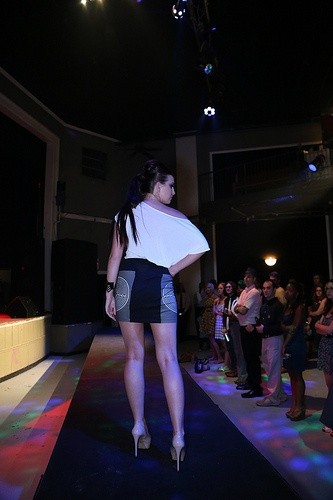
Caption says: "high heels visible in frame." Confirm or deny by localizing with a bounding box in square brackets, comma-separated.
[171, 434, 186, 471]
[292, 410, 305, 421]
[132, 419, 151, 456]
[286, 409, 295, 417]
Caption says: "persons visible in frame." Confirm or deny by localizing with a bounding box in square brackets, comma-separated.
[314, 279, 333, 437]
[175, 283, 190, 340]
[193, 272, 326, 422]
[234, 269, 263, 399]
[105, 159, 211, 471]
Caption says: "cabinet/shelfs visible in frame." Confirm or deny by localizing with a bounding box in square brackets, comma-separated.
[51, 322, 96, 353]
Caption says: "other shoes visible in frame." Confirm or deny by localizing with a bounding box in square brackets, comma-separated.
[257, 397, 280, 406]
[330, 430, 333, 437]
[322, 426, 332, 433]
[225, 371, 238, 377]
[219, 366, 230, 372]
[214, 359, 224, 364]
[234, 378, 247, 385]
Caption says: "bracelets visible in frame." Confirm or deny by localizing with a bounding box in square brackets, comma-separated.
[236, 307, 238, 313]
[106, 280, 116, 293]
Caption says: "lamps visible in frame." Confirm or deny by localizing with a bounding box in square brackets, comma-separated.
[308, 154, 325, 172]
[200, 63, 216, 120]
[172, 0, 188, 19]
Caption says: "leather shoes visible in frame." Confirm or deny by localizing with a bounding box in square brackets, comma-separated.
[236, 384, 249, 390]
[242, 390, 263, 398]
